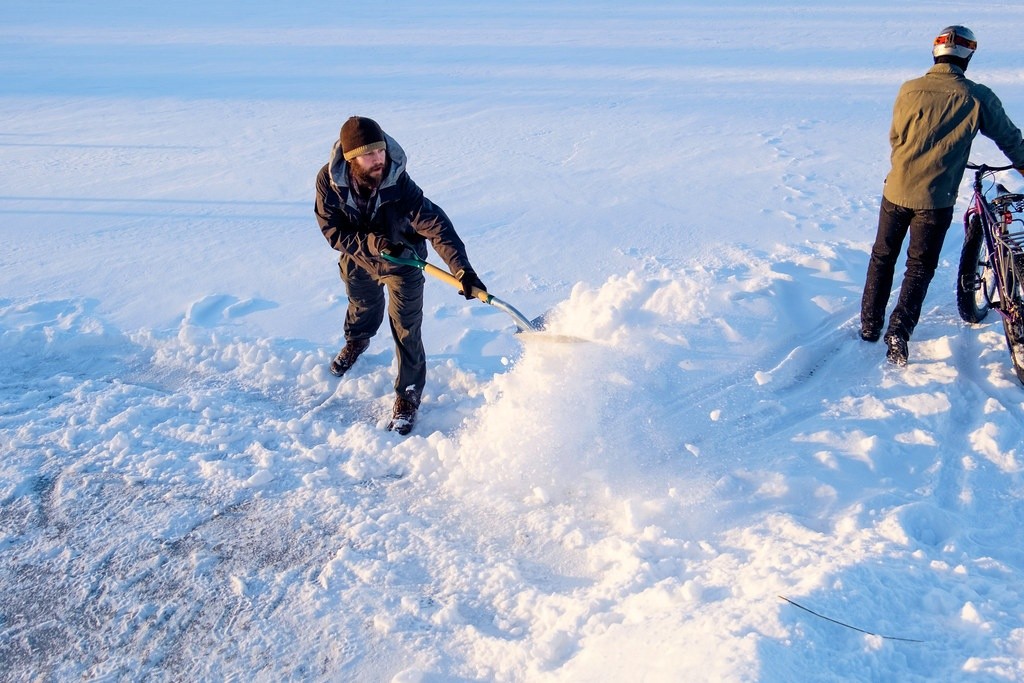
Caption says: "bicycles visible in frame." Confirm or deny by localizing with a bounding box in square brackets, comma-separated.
[957, 161, 1024, 385]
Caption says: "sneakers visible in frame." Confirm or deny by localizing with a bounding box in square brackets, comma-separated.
[329, 338, 370, 377]
[387, 395, 417, 435]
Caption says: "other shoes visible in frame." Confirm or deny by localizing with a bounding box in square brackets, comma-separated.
[862, 328, 880, 341]
[884, 331, 909, 367]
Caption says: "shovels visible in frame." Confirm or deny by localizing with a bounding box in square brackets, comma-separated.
[380, 241, 554, 343]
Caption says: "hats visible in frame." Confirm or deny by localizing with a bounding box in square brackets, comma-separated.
[339, 116, 386, 161]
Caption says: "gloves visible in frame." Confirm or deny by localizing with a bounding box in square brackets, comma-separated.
[373, 236, 405, 262]
[454, 269, 487, 300]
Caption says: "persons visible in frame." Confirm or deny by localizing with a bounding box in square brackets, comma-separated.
[860, 25, 1024, 364]
[314, 116, 486, 434]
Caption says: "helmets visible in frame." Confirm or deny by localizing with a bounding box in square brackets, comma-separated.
[932, 25, 977, 59]
